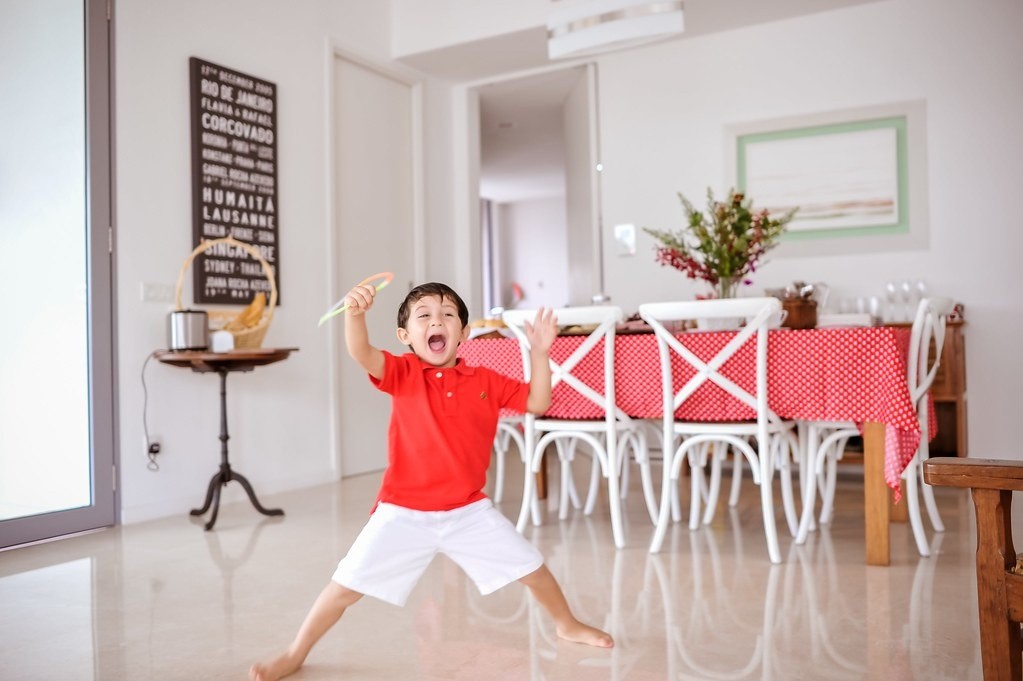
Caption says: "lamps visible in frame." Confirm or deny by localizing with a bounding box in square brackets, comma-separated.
[548, 0, 684, 61]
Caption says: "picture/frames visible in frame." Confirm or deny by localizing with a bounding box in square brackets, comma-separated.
[722, 101, 929, 253]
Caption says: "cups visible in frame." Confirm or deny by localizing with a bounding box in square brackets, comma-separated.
[785, 300, 817, 329]
[745, 301, 788, 328]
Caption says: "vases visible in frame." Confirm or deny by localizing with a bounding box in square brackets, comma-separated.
[715, 274, 740, 301]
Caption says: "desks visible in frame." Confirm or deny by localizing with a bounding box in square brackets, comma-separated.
[155, 347, 295, 530]
[462, 326, 936, 565]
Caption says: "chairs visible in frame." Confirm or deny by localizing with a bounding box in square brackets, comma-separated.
[486, 295, 945, 557]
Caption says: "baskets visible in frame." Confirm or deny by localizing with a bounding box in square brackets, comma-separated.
[176, 233, 277, 350]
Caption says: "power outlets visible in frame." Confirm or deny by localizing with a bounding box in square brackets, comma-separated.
[145, 436, 160, 457]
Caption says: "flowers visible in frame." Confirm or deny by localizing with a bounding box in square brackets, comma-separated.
[641, 183, 797, 282]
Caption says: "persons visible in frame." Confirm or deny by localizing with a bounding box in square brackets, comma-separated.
[251, 283, 616, 681]
